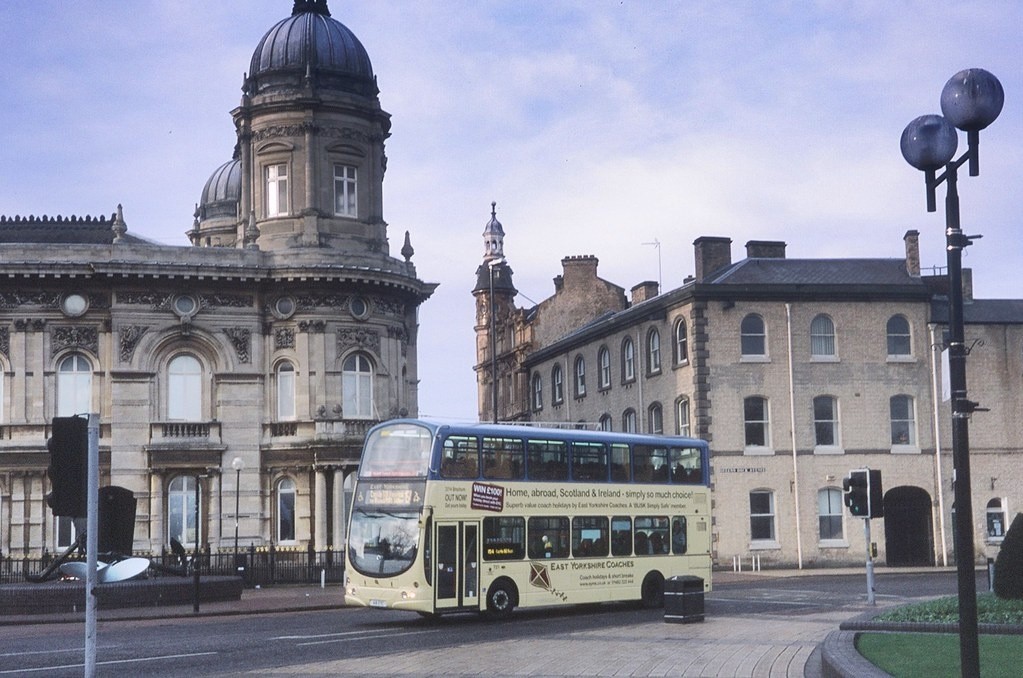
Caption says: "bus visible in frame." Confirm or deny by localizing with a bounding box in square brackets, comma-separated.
[341, 419, 716, 622]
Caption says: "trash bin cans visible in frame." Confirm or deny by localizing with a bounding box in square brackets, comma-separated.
[663, 575, 707, 625]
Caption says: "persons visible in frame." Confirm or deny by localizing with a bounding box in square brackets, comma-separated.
[673, 520, 685, 546]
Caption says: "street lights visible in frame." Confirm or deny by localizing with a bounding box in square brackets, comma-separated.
[232, 457, 244, 577]
[490, 255, 506, 425]
[897, 66, 1009, 678]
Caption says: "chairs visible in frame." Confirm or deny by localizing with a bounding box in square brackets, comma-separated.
[440, 458, 704, 484]
[576, 533, 687, 556]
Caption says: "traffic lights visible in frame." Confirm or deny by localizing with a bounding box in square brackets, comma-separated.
[848, 470, 871, 517]
